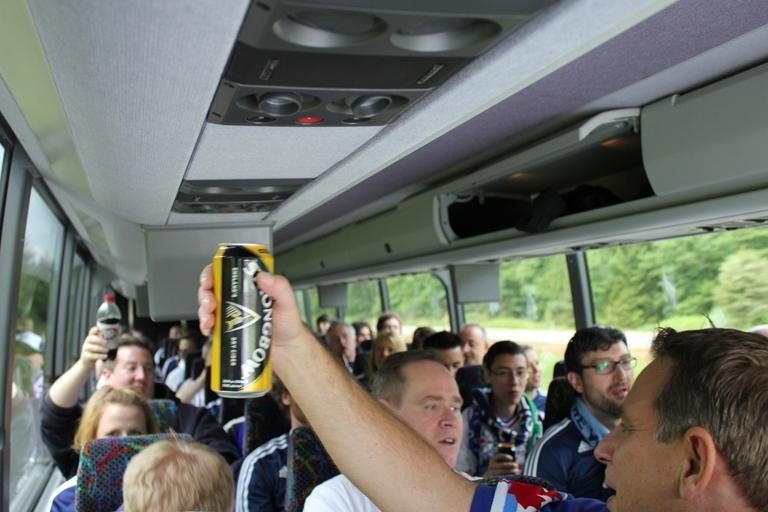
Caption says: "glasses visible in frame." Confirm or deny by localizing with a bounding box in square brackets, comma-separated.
[581, 357, 637, 376]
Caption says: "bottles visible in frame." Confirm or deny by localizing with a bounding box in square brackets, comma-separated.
[96, 293, 122, 362]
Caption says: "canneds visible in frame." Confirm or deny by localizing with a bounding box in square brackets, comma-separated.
[210, 244, 275, 400]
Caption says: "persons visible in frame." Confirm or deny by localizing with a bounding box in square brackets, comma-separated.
[39, 312, 639, 512]
[194, 259, 768, 512]
[9, 313, 44, 505]
